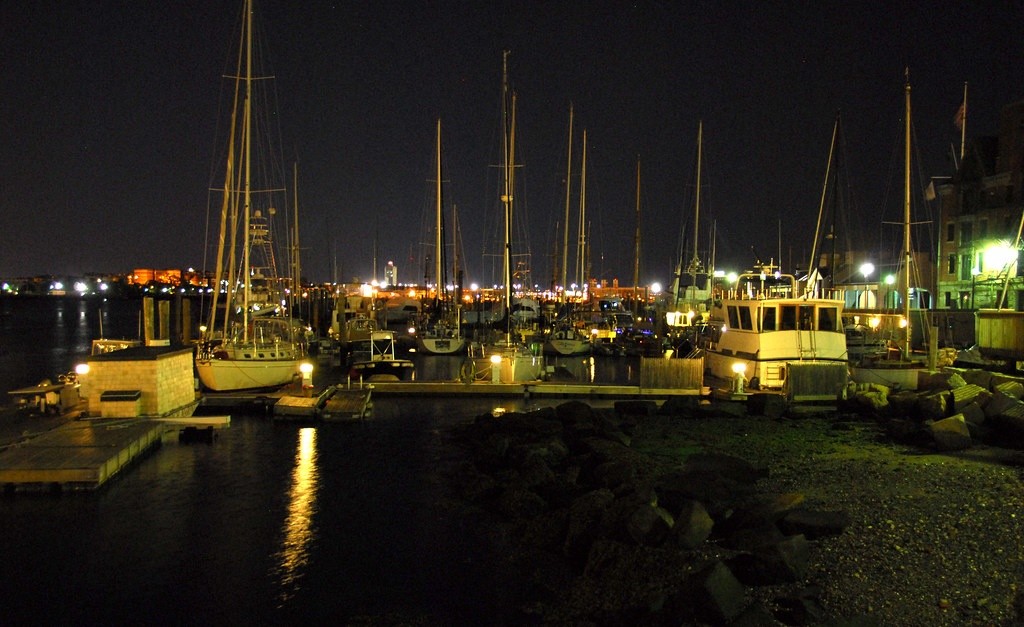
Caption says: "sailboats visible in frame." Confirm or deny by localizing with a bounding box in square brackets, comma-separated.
[195, 0, 311, 391]
[853, 66, 945, 392]
[473, 49, 717, 384]
[417, 117, 465, 354]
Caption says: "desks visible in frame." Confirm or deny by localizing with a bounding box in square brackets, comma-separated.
[8, 385, 65, 415]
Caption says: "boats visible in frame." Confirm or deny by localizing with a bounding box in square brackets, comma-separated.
[339, 312, 419, 381]
[0, 374, 88, 446]
[704, 257, 851, 391]
